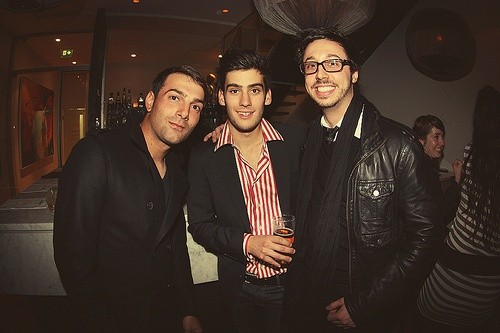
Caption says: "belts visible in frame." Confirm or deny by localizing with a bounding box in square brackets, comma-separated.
[243, 273, 287, 287]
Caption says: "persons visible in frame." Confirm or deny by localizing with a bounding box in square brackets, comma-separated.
[203, 27, 437, 333]
[412, 114, 463, 221]
[187, 49, 301, 333]
[415, 86, 500, 333]
[53, 65, 209, 333]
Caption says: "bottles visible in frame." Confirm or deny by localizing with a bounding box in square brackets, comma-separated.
[116, 92, 121, 108]
[108, 93, 114, 108]
[122, 88, 126, 107]
[127, 90, 131, 108]
[138, 93, 144, 108]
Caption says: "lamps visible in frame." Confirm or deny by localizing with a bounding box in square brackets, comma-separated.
[252, 1, 377, 38]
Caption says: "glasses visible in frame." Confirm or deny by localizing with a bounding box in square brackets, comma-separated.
[299, 57, 356, 75]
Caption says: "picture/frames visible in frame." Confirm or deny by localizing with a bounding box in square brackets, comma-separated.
[18, 76, 56, 170]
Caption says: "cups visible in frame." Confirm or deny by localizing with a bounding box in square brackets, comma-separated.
[271, 214, 296, 269]
